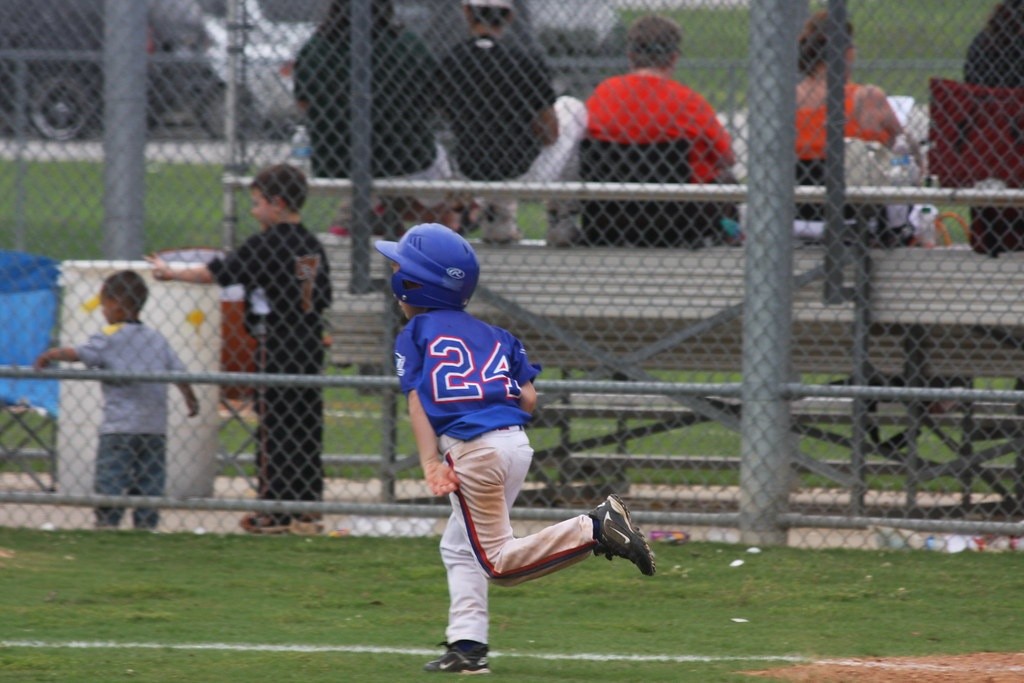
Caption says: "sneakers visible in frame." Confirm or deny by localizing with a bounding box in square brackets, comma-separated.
[588, 494, 656, 576]
[423, 642, 491, 675]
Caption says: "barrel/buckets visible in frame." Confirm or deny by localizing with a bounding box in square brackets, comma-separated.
[58, 260, 221, 498]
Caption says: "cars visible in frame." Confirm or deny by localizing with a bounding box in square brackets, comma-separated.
[0, 0, 301, 141]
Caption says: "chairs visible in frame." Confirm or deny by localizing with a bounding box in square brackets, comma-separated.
[0, 249, 62, 492]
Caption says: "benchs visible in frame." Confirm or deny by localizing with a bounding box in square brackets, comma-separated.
[242, 171, 1024, 528]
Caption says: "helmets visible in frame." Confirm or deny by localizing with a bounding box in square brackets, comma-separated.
[374, 223, 480, 311]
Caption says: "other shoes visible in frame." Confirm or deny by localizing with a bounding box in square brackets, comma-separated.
[290, 517, 324, 537]
[479, 213, 521, 244]
[94, 521, 118, 532]
[239, 514, 291, 534]
[328, 206, 386, 246]
[546, 220, 583, 249]
[136, 519, 157, 533]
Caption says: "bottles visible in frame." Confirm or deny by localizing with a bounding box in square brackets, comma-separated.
[291, 126, 310, 175]
[893, 135, 909, 174]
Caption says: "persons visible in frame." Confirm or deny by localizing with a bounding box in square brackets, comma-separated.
[375, 221, 655, 672]
[141, 162, 332, 535]
[433, 0, 587, 246]
[34, 270, 201, 531]
[292, 0, 482, 235]
[585, 14, 742, 249]
[797, 0, 1023, 253]
